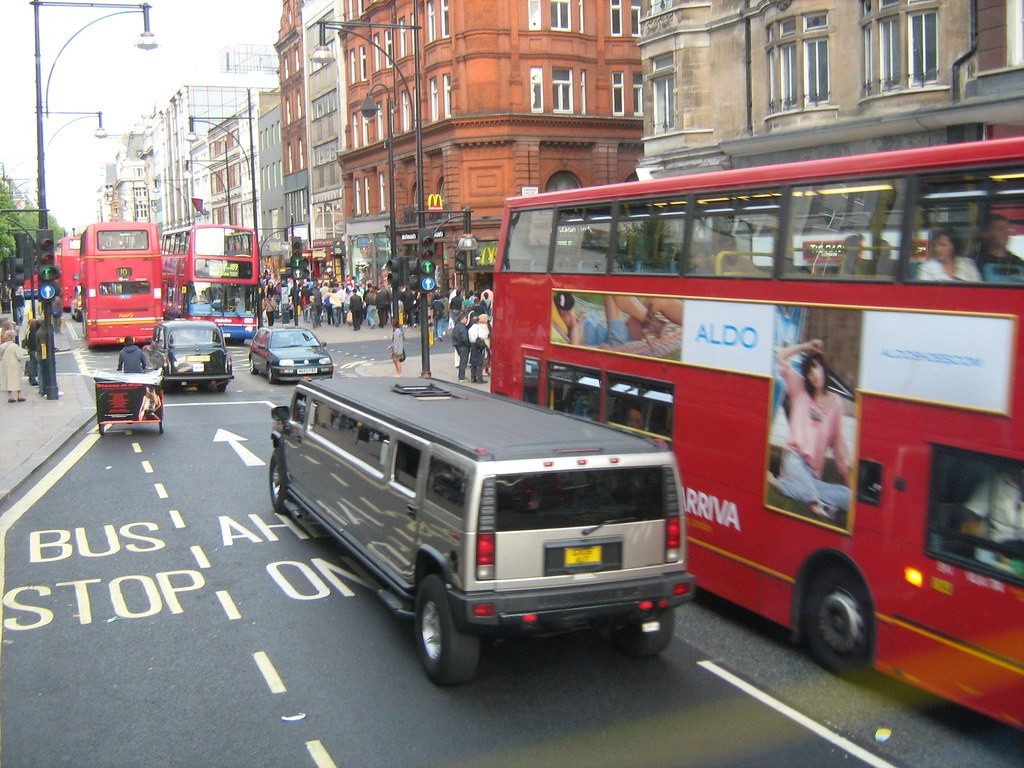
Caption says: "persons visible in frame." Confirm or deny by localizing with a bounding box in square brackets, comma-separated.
[0, 315, 26, 403]
[960, 464, 1024, 545]
[970, 214, 1024, 277]
[16, 289, 25, 326]
[431, 290, 491, 384]
[626, 406, 646, 430]
[767, 339, 850, 519]
[116, 336, 147, 373]
[53, 298, 63, 335]
[845, 234, 890, 275]
[191, 290, 208, 304]
[693, 235, 738, 274]
[387, 320, 404, 375]
[266, 277, 420, 331]
[24, 312, 64, 397]
[553, 292, 683, 346]
[917, 228, 983, 283]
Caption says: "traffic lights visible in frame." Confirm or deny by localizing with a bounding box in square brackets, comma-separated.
[408, 256, 420, 290]
[10, 258, 25, 290]
[417, 227, 438, 294]
[36, 228, 58, 303]
[386, 257, 410, 287]
[454, 247, 467, 271]
[289, 235, 310, 283]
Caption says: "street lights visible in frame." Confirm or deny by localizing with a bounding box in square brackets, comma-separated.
[181, 141, 237, 224]
[183, 87, 263, 328]
[311, 1, 435, 376]
[359, 83, 403, 329]
[30, 0, 162, 399]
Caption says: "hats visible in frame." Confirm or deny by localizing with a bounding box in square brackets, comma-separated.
[553, 291, 575, 310]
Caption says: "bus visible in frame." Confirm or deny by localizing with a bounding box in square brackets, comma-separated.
[489, 134, 1024, 739]
[160, 224, 260, 342]
[77, 221, 164, 347]
[54, 235, 85, 312]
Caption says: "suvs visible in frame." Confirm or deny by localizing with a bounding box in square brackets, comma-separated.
[265, 375, 696, 687]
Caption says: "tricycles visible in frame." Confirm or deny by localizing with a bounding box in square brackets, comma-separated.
[94, 369, 166, 436]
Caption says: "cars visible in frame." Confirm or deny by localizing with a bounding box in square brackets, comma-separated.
[249, 327, 334, 383]
[69, 286, 83, 322]
[147, 320, 235, 393]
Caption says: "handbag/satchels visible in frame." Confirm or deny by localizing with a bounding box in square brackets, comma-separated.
[475, 337, 486, 353]
[347, 310, 353, 326]
[400, 350, 406, 362]
[22, 339, 27, 348]
[24, 361, 38, 377]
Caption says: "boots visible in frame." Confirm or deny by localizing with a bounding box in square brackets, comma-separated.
[642, 312, 668, 339]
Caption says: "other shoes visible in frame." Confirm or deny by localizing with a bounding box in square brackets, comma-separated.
[18, 398, 27, 402]
[413, 324, 416, 328]
[335, 322, 339, 327]
[8, 399, 17, 403]
[438, 336, 443, 342]
[459, 379, 469, 383]
[370, 323, 375, 329]
[403, 325, 408, 328]
[478, 379, 487, 383]
[31, 383, 39, 386]
[471, 379, 477, 383]
